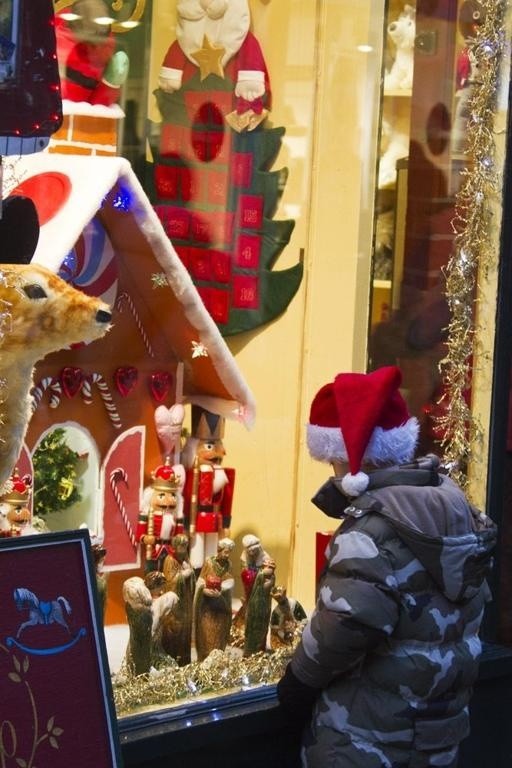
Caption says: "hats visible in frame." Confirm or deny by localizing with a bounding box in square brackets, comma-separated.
[306, 364, 420, 499]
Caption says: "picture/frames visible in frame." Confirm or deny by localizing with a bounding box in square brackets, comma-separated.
[0, 527, 126, 767]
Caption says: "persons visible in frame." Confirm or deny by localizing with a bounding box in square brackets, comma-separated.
[178, 404, 232, 577]
[277, 367, 496, 767]
[138, 464, 189, 573]
[1, 476, 32, 539]
[91, 533, 307, 677]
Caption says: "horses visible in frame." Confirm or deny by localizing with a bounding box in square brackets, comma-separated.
[10, 586, 74, 640]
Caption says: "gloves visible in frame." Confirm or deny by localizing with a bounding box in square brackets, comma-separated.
[277, 662, 318, 735]
